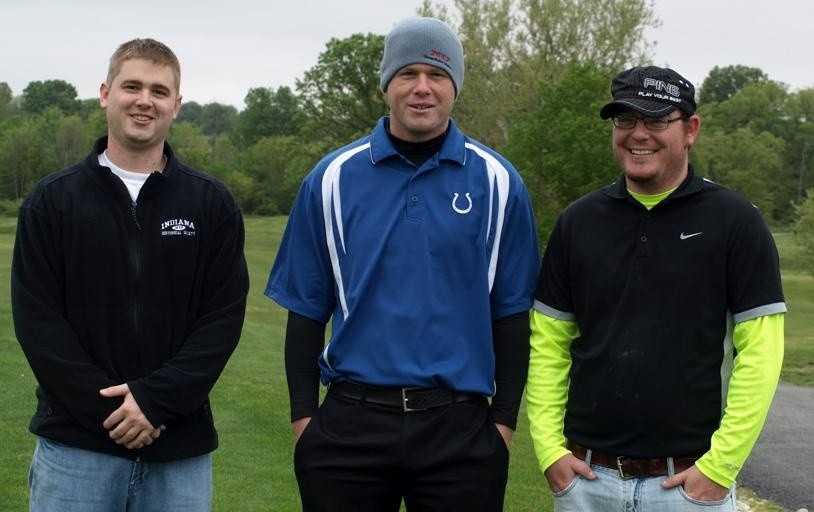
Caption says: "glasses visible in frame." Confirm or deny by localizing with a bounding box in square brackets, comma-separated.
[612, 113, 685, 130]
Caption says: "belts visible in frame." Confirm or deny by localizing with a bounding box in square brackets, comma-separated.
[329, 378, 484, 412]
[566, 438, 698, 481]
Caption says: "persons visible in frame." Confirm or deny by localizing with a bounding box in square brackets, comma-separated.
[524, 64, 787, 511]
[264, 17, 542, 510]
[11, 37, 250, 512]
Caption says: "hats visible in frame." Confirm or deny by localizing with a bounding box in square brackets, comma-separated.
[380, 17, 465, 102]
[600, 66, 696, 120]
